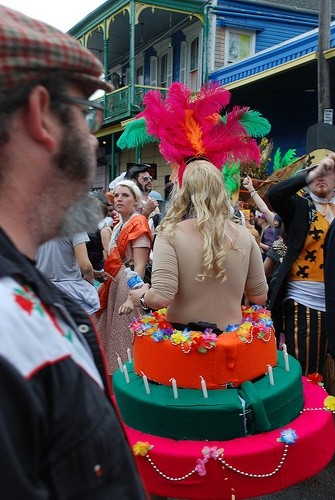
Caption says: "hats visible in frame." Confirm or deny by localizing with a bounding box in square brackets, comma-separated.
[0, 4, 115, 93]
[126, 163, 151, 179]
[295, 122, 335, 175]
[91, 190, 112, 207]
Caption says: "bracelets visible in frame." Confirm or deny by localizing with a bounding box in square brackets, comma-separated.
[313, 168, 322, 177]
[251, 191, 257, 198]
[249, 189, 256, 194]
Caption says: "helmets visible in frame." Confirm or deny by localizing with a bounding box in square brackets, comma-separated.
[149, 191, 164, 202]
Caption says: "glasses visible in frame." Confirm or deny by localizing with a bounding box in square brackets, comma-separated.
[142, 176, 153, 181]
[258, 216, 262, 219]
[60, 95, 104, 134]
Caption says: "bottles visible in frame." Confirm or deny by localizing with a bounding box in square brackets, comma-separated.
[124, 267, 151, 310]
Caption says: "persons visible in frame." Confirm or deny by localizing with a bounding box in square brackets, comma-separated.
[36, 123, 335, 500]
[0, 6, 147, 500]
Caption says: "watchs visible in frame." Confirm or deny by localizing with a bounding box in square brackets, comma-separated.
[140, 292, 148, 310]
